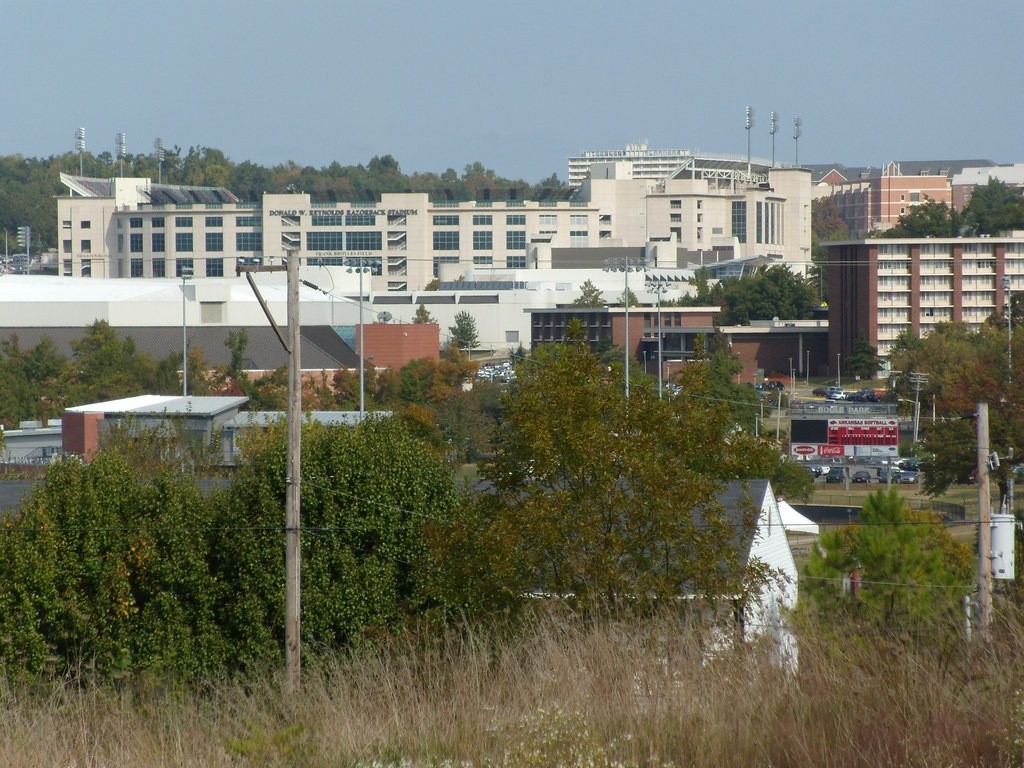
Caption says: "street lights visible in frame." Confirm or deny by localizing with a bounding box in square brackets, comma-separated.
[345, 256, 386, 429]
[179, 264, 195, 397]
[898, 398, 921, 442]
[601, 252, 644, 412]
[643, 276, 673, 404]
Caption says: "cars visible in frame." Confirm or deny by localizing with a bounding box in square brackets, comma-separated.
[804, 463, 830, 477]
[873, 455, 925, 485]
[746, 379, 814, 409]
[814, 386, 886, 403]
[852, 470, 872, 484]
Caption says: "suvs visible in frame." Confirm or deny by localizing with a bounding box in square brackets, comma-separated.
[825, 466, 844, 484]
